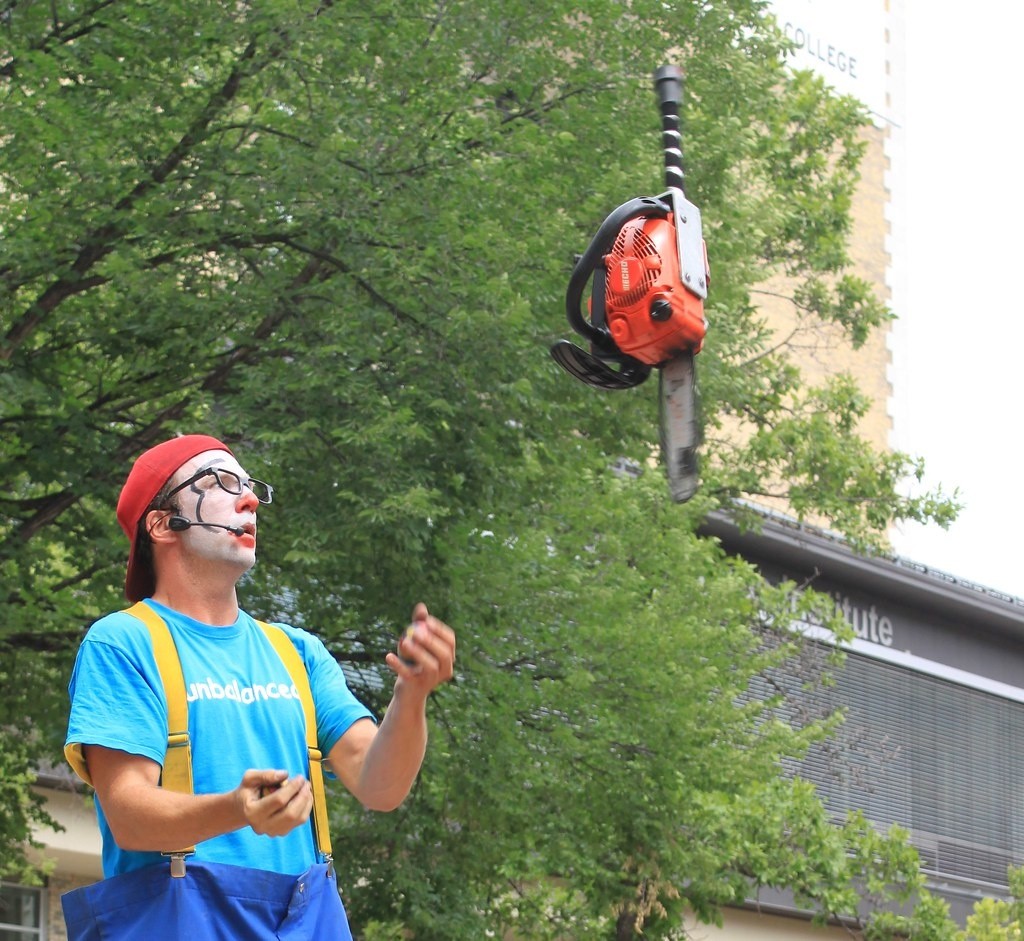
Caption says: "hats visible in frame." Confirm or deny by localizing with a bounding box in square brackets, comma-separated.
[117, 435, 234, 603]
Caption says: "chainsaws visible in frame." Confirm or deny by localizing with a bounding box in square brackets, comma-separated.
[548, 64, 709, 504]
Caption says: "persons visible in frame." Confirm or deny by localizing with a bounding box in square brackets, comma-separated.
[61, 436, 455, 941]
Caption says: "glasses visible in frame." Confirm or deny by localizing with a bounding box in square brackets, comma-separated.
[157, 467, 275, 508]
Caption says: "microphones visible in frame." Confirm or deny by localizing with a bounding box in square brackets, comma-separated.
[170, 515, 245, 536]
